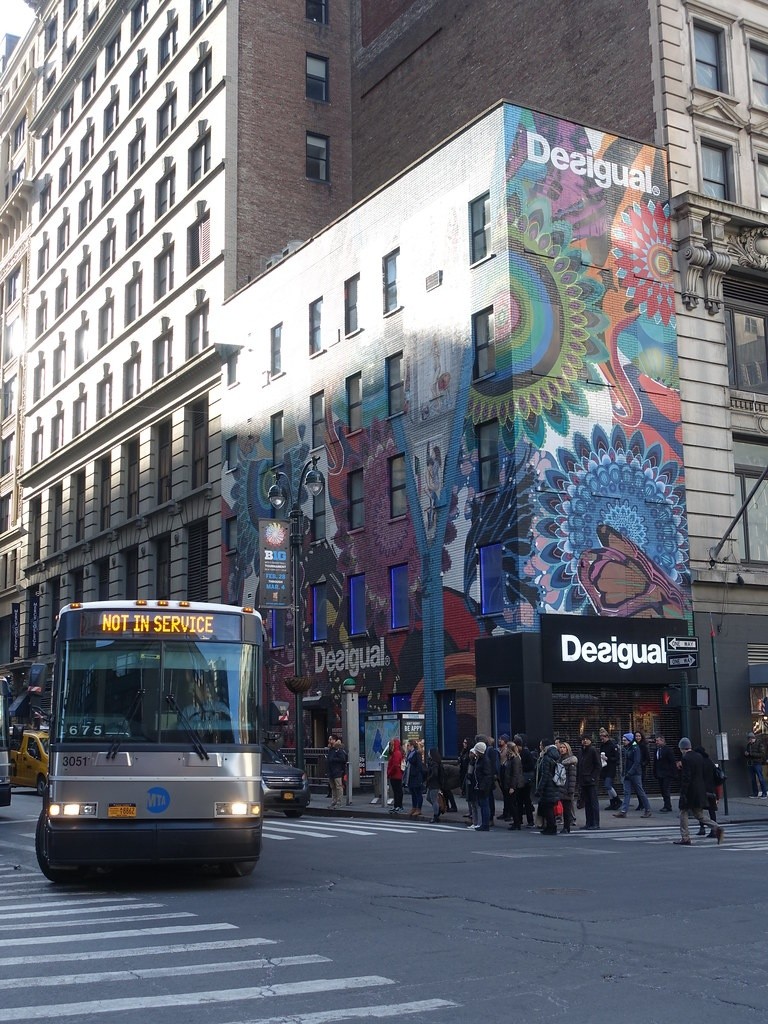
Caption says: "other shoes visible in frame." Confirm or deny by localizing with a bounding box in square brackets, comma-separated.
[659, 805, 672, 813]
[386, 801, 579, 835]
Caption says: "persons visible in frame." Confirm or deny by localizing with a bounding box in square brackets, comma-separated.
[324, 737, 347, 797]
[426, 748, 445, 824]
[404, 739, 425, 817]
[324, 734, 346, 808]
[442, 764, 462, 813]
[174, 679, 238, 744]
[673, 737, 725, 845]
[387, 738, 405, 814]
[456, 728, 652, 836]
[744, 731, 768, 800]
[652, 734, 674, 813]
[692, 744, 719, 839]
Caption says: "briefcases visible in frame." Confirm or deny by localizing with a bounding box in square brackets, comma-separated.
[700, 792, 719, 810]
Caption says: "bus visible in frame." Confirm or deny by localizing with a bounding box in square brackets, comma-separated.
[36, 598, 269, 886]
[0, 675, 12, 808]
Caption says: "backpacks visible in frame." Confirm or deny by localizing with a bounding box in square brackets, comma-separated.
[549, 756, 566, 786]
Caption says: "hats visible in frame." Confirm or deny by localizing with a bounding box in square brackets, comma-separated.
[581, 733, 590, 740]
[678, 737, 692, 748]
[474, 742, 486, 754]
[470, 747, 477, 757]
[500, 733, 510, 743]
[623, 733, 634, 742]
[747, 732, 756, 739]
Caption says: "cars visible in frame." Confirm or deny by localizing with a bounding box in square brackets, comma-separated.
[361, 756, 374, 783]
[9, 726, 50, 796]
[259, 742, 311, 819]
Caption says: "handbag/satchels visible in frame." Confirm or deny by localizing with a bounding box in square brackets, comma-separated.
[523, 771, 535, 784]
[713, 767, 727, 786]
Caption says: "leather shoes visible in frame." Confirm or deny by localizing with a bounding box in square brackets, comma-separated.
[715, 826, 724, 846]
[587, 824, 600, 830]
[579, 824, 589, 830]
[673, 837, 691, 846]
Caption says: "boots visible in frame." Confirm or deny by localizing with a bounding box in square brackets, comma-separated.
[613, 793, 622, 810]
[707, 829, 716, 837]
[326, 784, 332, 798]
[635, 795, 645, 811]
[603, 798, 617, 810]
[695, 827, 705, 835]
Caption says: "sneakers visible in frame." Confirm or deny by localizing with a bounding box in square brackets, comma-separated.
[640, 811, 651, 818]
[326, 800, 337, 809]
[748, 792, 758, 798]
[759, 792, 767, 799]
[612, 812, 627, 817]
[330, 802, 342, 810]
